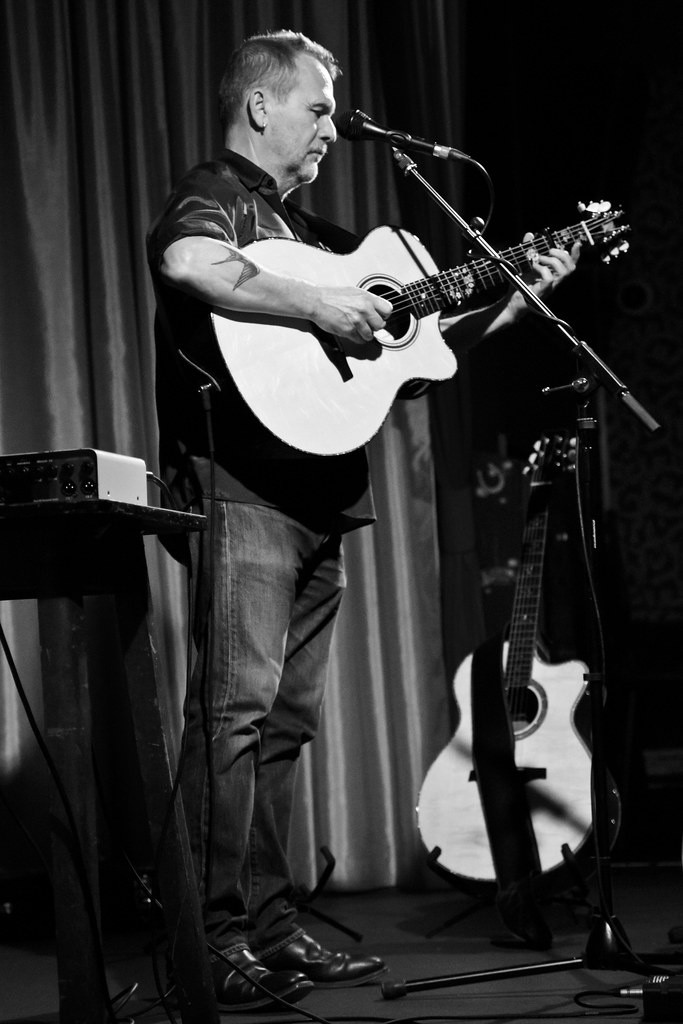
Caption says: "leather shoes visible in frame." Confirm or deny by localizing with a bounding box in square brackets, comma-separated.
[176, 932, 385, 1011]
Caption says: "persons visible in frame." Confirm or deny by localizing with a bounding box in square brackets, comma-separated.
[145, 27, 584, 1010]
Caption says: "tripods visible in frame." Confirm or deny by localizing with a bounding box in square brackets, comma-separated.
[381, 373, 677, 1002]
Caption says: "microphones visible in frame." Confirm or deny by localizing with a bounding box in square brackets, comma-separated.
[335, 107, 473, 163]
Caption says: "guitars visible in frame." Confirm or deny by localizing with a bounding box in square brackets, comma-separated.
[209, 199, 630, 459]
[414, 433, 622, 902]
